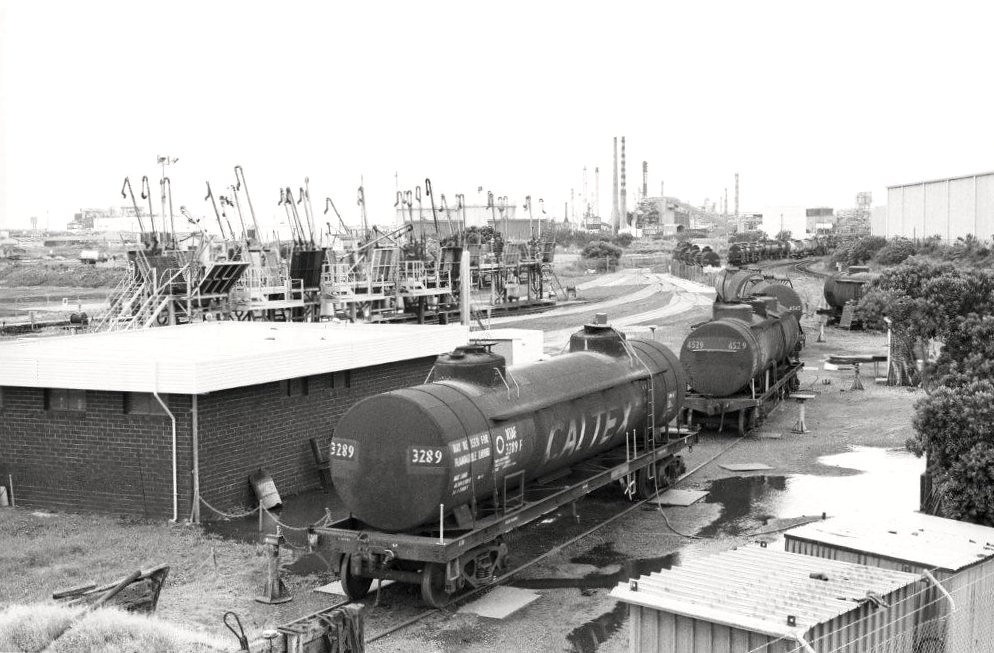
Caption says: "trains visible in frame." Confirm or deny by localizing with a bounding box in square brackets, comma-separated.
[294, 266, 810, 610]
